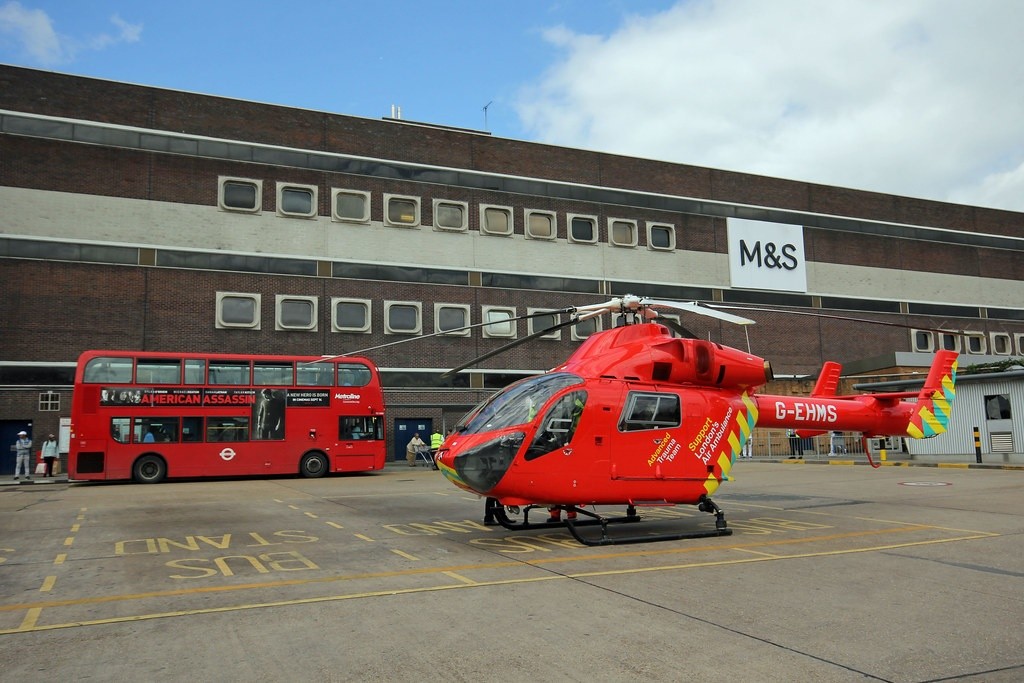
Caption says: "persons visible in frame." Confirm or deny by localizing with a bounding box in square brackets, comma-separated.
[785, 428, 804, 460]
[256, 389, 284, 439]
[405, 433, 426, 467]
[352, 422, 365, 437]
[40, 433, 60, 477]
[430, 429, 445, 470]
[741, 430, 753, 458]
[142, 426, 159, 441]
[12, 431, 32, 479]
[102, 388, 143, 406]
[445, 429, 452, 437]
[828, 430, 847, 456]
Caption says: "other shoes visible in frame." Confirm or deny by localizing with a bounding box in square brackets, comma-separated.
[749, 457, 753, 459]
[13, 477, 19, 480]
[798, 456, 802, 459]
[408, 465, 414, 467]
[43, 474, 46, 477]
[25, 476, 30, 480]
[49, 474, 54, 477]
[433, 466, 438, 471]
[788, 456, 796, 459]
[742, 456, 746, 459]
[413, 465, 416, 466]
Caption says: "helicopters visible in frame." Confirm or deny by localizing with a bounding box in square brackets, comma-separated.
[302, 294, 985, 545]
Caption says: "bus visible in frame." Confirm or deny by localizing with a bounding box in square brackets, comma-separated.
[67, 350, 386, 484]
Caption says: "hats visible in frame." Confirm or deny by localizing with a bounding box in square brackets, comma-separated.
[17, 431, 27, 435]
